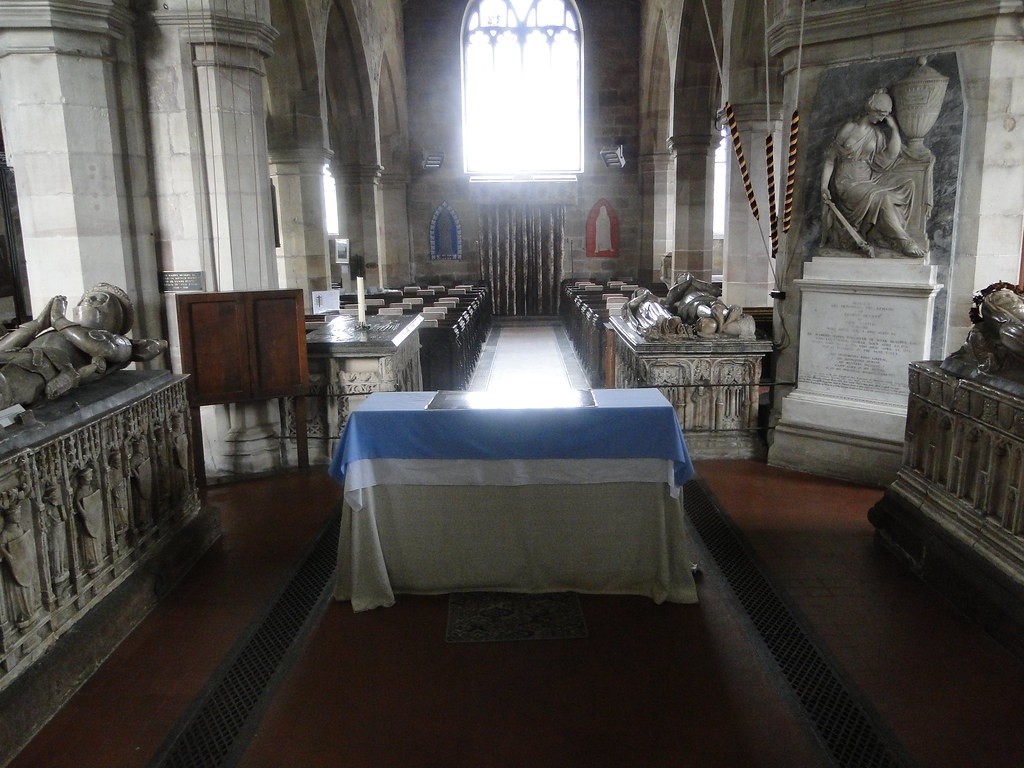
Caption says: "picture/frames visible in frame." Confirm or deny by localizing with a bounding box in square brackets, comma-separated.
[334, 239, 349, 263]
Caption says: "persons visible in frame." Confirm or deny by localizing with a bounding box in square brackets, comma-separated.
[977, 287, 1024, 361]
[0, 411, 193, 634]
[820, 87, 926, 258]
[621, 287, 683, 335]
[666, 271, 756, 335]
[0, 282, 135, 411]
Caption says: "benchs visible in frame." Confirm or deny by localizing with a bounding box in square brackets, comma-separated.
[305, 283, 493, 390]
[559, 277, 773, 391]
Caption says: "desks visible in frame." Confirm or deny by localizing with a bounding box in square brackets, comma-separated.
[352, 388, 675, 596]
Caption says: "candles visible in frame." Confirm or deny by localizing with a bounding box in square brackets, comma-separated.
[357, 276, 366, 322]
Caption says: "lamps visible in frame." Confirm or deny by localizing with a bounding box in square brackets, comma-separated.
[422, 147, 445, 170]
[600, 137, 627, 169]
